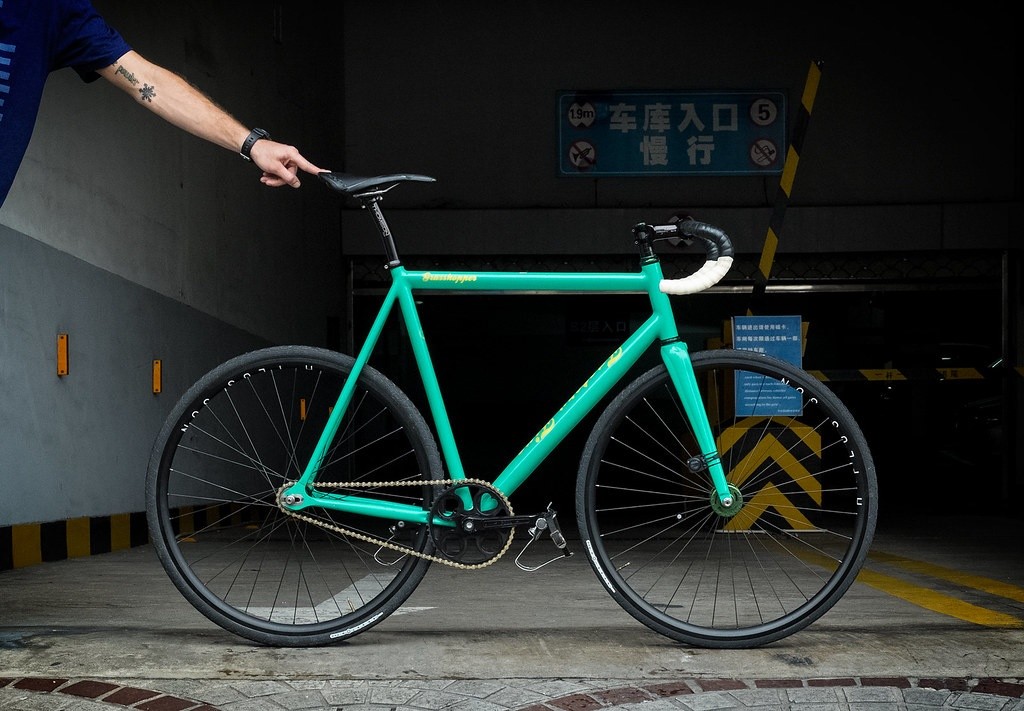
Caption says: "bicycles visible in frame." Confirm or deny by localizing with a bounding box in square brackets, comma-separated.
[143, 169, 879, 648]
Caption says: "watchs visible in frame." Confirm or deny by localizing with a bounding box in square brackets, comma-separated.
[240, 127, 272, 161]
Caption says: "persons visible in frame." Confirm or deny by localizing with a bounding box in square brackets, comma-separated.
[0, 0, 334, 207]
